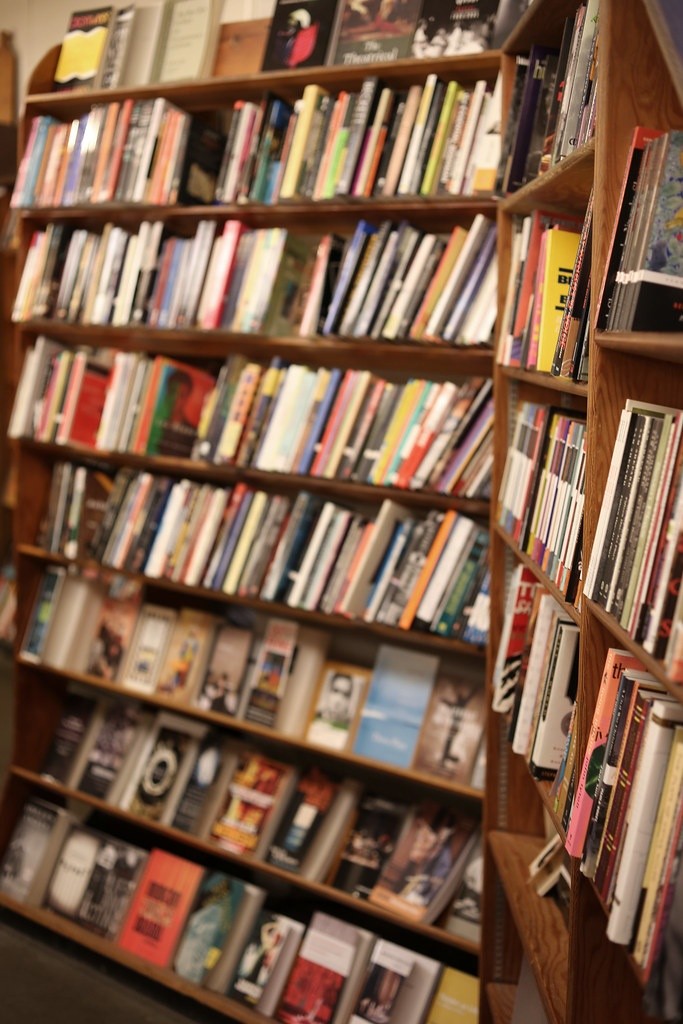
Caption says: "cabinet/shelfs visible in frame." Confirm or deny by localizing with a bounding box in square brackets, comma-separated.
[477, 0, 682, 1024]
[1, 50, 517, 1024]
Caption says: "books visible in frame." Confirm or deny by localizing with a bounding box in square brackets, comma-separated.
[0, 0, 683, 1024]
